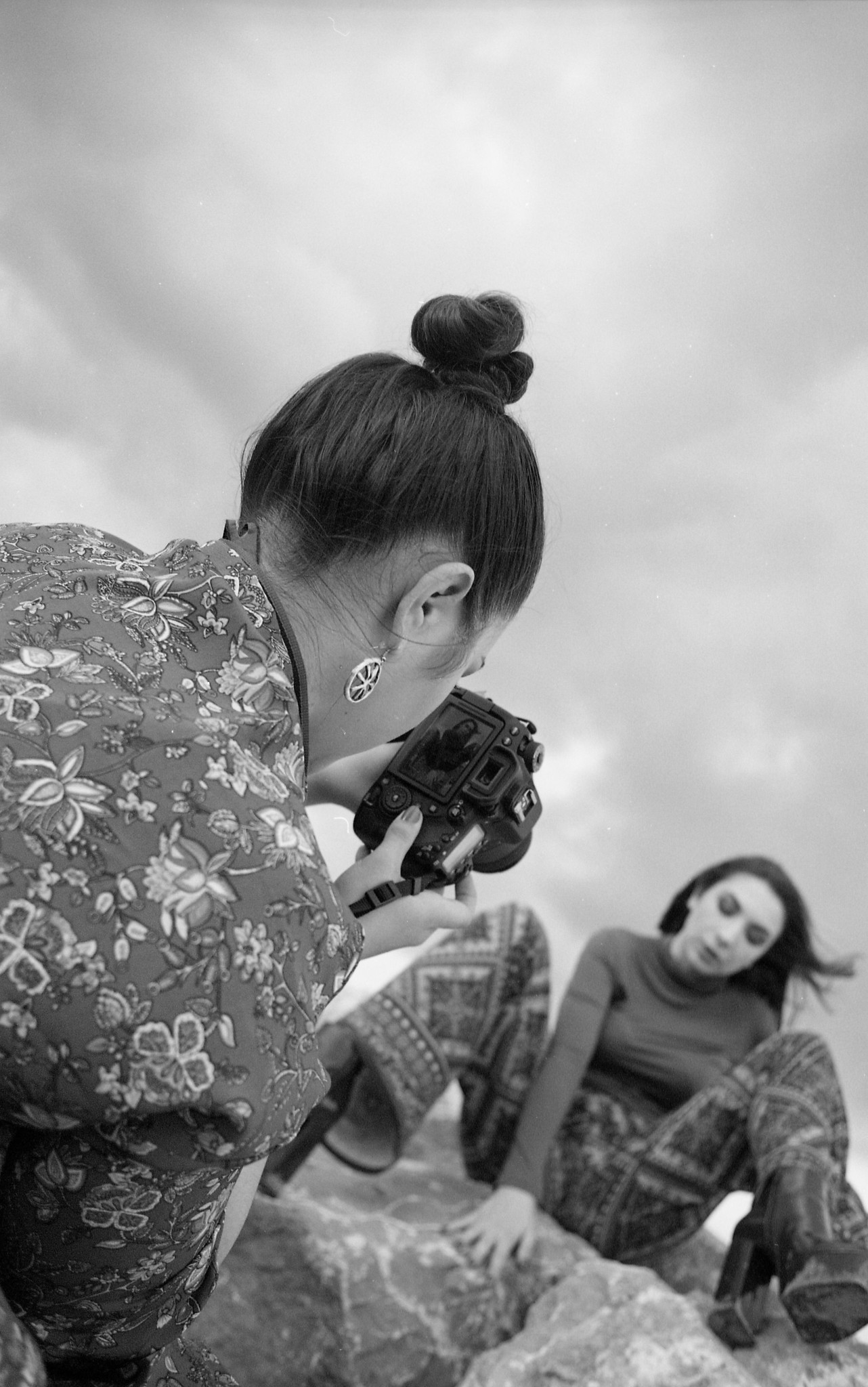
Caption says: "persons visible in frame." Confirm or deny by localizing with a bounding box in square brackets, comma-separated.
[260, 854, 867, 1346]
[0, 292, 547, 1385]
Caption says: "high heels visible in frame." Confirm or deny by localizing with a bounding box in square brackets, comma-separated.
[255, 1023, 364, 1199]
[705, 1166, 868, 1347]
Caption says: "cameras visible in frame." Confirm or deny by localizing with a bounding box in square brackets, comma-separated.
[354, 686, 544, 884]
